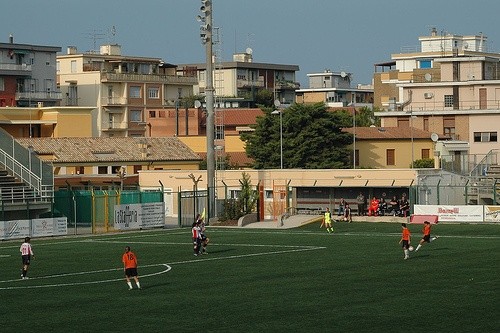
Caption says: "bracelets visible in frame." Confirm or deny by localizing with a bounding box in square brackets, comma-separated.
[400, 240, 402, 243]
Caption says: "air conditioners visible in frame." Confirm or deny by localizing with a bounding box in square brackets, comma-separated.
[424, 92, 431, 99]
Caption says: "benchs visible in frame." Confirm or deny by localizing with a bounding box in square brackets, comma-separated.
[299, 209, 312, 215]
[0, 164, 40, 201]
[463, 165, 500, 196]
[313, 210, 324, 215]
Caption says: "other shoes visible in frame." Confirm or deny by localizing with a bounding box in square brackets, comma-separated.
[21, 274, 29, 279]
[404, 257, 409, 259]
[435, 236, 440, 239]
[415, 250, 418, 252]
[199, 251, 202, 255]
[194, 253, 198, 256]
[202, 251, 208, 254]
[129, 287, 133, 291]
[202, 247, 207, 251]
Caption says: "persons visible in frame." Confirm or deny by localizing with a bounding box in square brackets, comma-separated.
[338, 197, 347, 216]
[20, 237, 34, 279]
[412, 221, 440, 253]
[190, 213, 210, 256]
[122, 246, 144, 290]
[322, 208, 335, 234]
[340, 204, 352, 223]
[367, 195, 409, 217]
[398, 223, 411, 260]
[356, 192, 365, 216]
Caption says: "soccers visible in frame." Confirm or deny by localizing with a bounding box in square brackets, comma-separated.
[408, 246, 414, 252]
[344, 218, 348, 221]
